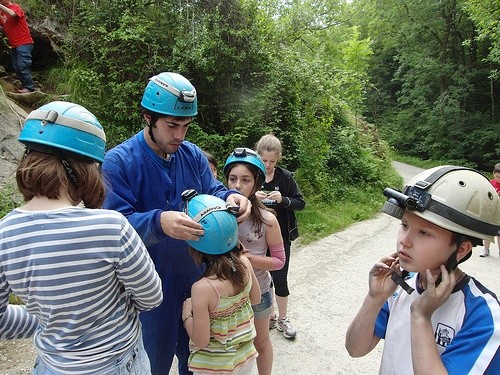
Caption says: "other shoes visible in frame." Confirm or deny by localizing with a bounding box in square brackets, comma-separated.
[18, 89, 29, 93]
[480, 249, 489, 257]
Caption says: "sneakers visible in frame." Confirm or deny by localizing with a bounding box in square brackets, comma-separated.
[269, 314, 276, 330]
[277, 317, 295, 338]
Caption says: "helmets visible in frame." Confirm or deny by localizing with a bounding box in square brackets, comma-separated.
[182, 194, 239, 255]
[17, 99, 106, 163]
[223, 148, 266, 184]
[140, 72, 197, 116]
[404, 164, 500, 242]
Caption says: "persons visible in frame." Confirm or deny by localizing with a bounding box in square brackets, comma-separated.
[98, 72, 253, 375]
[345, 165, 500, 375]
[255, 135, 305, 338]
[204, 152, 217, 180]
[0, 0, 36, 93]
[480, 163, 500, 257]
[224, 148, 286, 374]
[0, 101, 164, 375]
[180, 189, 261, 375]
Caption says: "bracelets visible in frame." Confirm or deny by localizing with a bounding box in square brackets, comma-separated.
[183, 315, 192, 324]
